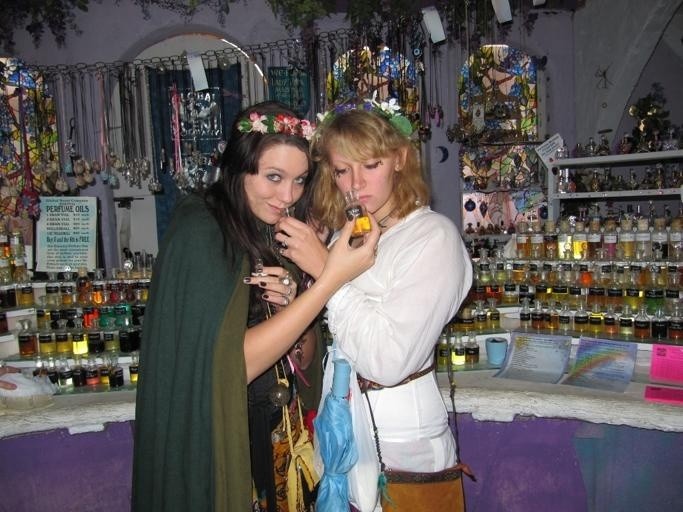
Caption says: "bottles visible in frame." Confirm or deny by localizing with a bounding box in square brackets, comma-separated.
[556, 128, 683, 216]
[436, 217, 682, 334]
[436, 333, 450, 371]
[9, 230, 21, 247]
[0, 246, 15, 333]
[450, 333, 465, 365]
[15, 247, 156, 395]
[464, 331, 479, 364]
[279, 208, 295, 217]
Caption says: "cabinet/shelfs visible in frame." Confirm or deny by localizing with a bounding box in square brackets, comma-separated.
[0, 257, 683, 375]
[546, 144, 682, 229]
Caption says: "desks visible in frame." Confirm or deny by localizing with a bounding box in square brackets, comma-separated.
[0, 370, 683, 510]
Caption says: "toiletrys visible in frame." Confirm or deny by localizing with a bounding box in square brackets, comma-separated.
[463, 218, 680, 259]
[463, 268, 680, 314]
[0, 231, 155, 391]
[464, 247, 682, 287]
[435, 332, 483, 369]
[452, 298, 681, 341]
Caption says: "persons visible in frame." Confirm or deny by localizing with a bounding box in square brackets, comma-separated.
[274, 109, 473, 511]
[152, 100, 384, 511]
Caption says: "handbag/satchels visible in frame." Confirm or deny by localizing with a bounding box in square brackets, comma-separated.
[285, 430, 322, 512]
[379, 464, 476, 511]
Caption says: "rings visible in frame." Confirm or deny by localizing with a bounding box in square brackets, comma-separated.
[279, 235, 289, 249]
[278, 268, 293, 306]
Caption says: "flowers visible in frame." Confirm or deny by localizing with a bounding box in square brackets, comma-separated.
[311, 89, 417, 136]
[232, 113, 319, 141]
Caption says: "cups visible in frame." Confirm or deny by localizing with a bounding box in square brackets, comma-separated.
[485, 338, 508, 365]
